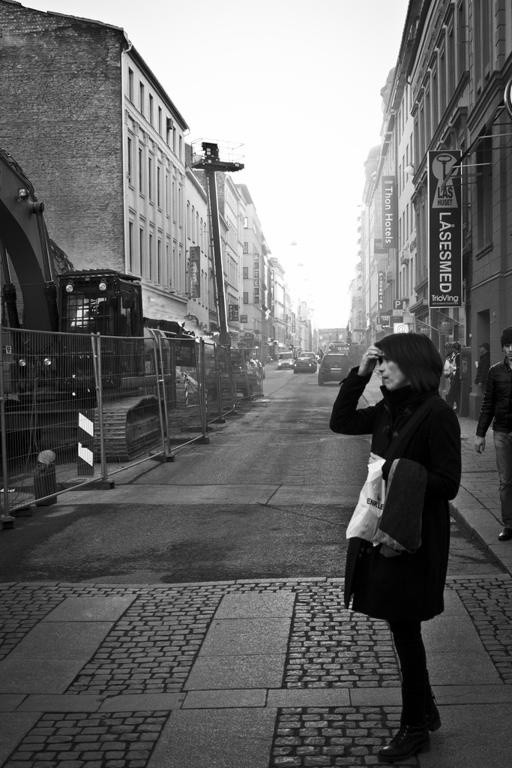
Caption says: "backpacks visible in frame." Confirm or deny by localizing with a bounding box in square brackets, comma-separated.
[443, 352, 460, 378]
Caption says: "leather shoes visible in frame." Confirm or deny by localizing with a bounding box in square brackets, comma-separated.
[378, 701, 442, 762]
[498, 527, 512, 541]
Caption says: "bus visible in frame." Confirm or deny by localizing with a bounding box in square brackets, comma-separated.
[320, 340, 353, 358]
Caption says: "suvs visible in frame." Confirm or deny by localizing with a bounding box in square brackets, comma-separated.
[318, 353, 354, 385]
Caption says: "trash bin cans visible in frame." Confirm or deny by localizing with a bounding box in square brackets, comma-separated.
[34, 450, 57, 506]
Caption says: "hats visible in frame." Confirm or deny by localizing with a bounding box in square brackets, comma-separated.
[479, 342, 490, 351]
[501, 327, 512, 347]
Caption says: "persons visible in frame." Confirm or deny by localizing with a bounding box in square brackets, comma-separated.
[330, 334, 461, 763]
[474, 326, 512, 542]
[444, 340, 464, 413]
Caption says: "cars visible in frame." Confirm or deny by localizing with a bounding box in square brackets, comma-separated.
[277, 346, 323, 375]
